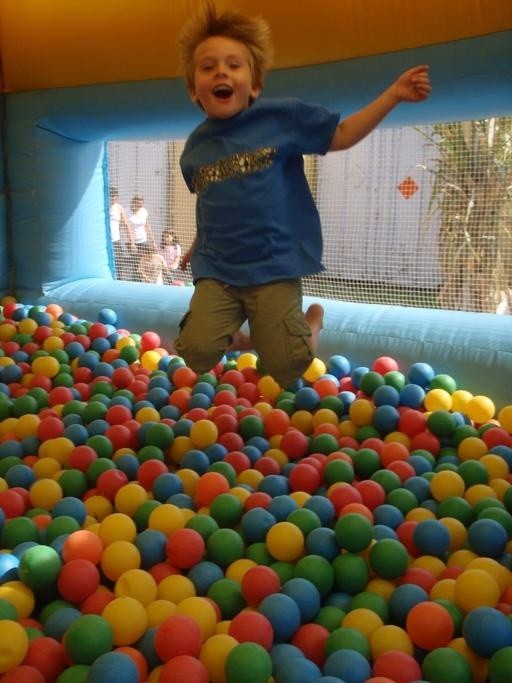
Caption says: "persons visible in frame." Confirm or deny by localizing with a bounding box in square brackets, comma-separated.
[119, 197, 158, 283]
[178, 0, 432, 389]
[157, 230, 181, 285]
[137, 242, 173, 284]
[108, 186, 138, 280]
